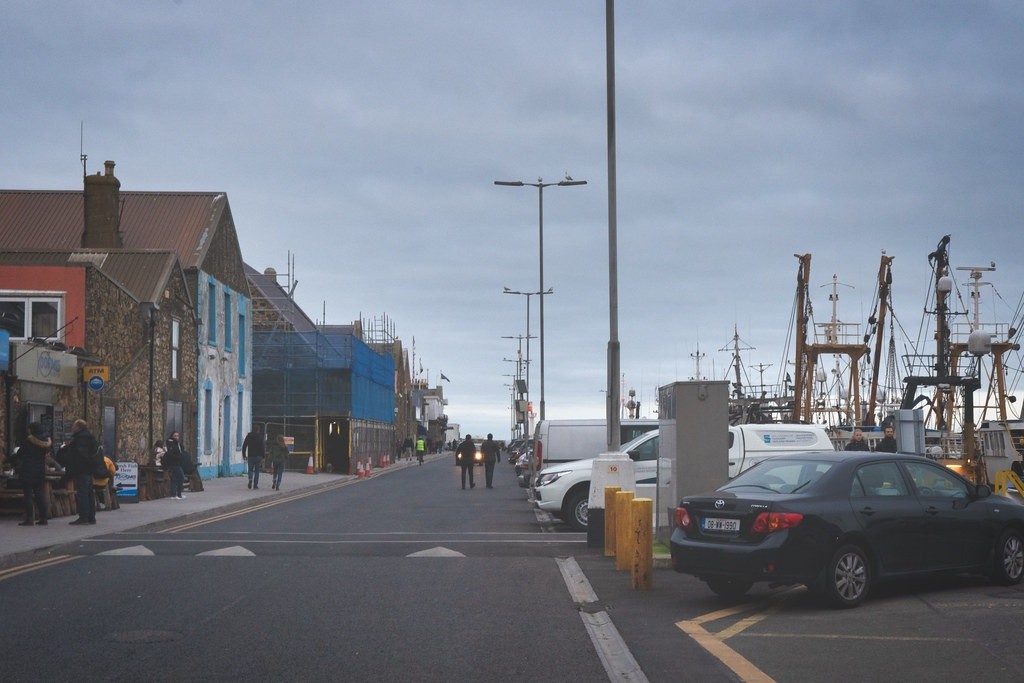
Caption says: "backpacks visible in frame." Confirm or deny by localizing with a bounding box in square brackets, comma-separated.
[148, 448, 158, 468]
[95, 459, 108, 479]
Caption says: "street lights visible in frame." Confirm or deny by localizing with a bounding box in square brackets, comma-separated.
[502, 359, 532, 438]
[493, 181, 587, 424]
[501, 336, 538, 439]
[502, 290, 553, 439]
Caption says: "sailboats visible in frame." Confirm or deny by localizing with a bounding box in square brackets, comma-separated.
[622, 235, 1021, 500]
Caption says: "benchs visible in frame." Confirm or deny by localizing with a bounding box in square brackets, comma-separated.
[0, 461, 205, 520]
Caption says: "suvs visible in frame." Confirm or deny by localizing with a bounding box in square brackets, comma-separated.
[535, 422, 837, 534]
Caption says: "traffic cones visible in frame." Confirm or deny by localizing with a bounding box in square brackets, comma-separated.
[355, 449, 393, 480]
[304, 453, 314, 474]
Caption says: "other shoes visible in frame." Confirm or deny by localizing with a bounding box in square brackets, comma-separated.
[169, 496, 182, 500]
[88, 517, 97, 524]
[396, 458, 426, 462]
[271, 484, 280, 491]
[471, 483, 476, 489]
[69, 517, 89, 525]
[486, 485, 493, 489]
[36, 519, 49, 525]
[247, 482, 259, 490]
[17, 516, 34, 526]
[461, 487, 465, 490]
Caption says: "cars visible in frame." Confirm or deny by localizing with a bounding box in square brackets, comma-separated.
[508, 438, 533, 489]
[670, 451, 1024, 609]
[456, 443, 484, 466]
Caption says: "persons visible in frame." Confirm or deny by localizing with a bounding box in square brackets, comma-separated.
[166, 432, 186, 498]
[481, 433, 501, 488]
[12, 442, 118, 489]
[845, 428, 870, 451]
[269, 434, 290, 491]
[64, 418, 98, 525]
[18, 423, 50, 526]
[396, 435, 456, 466]
[456, 434, 476, 489]
[876, 427, 897, 453]
[152, 440, 193, 473]
[495, 440, 511, 452]
[242, 424, 265, 488]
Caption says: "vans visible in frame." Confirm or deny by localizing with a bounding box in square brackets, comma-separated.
[534, 419, 662, 500]
